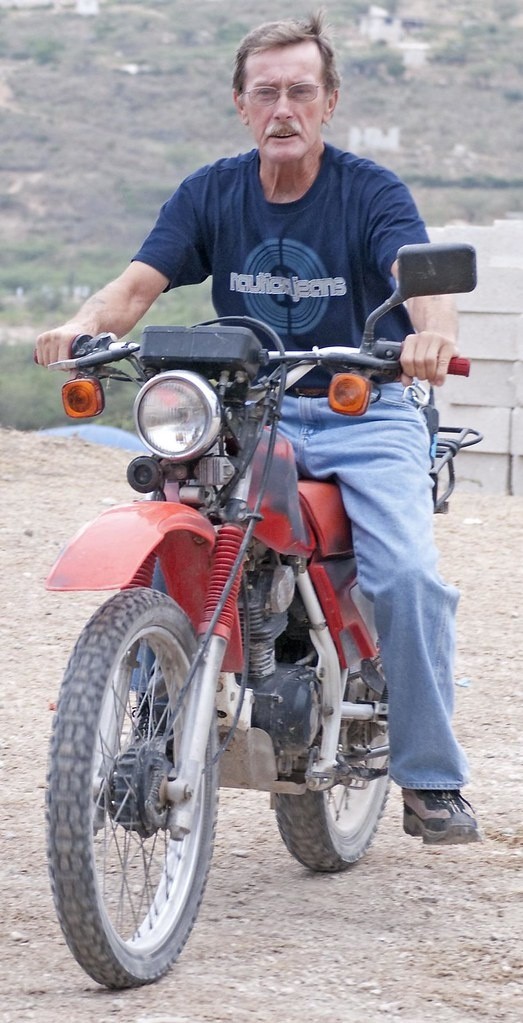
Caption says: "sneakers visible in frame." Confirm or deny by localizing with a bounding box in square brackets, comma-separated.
[401, 785, 486, 846]
[131, 692, 171, 752]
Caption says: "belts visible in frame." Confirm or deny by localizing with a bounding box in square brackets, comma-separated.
[287, 387, 330, 399]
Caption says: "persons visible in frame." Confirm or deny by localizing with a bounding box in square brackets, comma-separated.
[36, 7, 481, 844]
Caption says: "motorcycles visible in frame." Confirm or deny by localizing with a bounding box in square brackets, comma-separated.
[33, 241, 480, 992]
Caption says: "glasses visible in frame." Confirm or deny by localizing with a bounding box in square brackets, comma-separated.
[237, 82, 326, 107]
